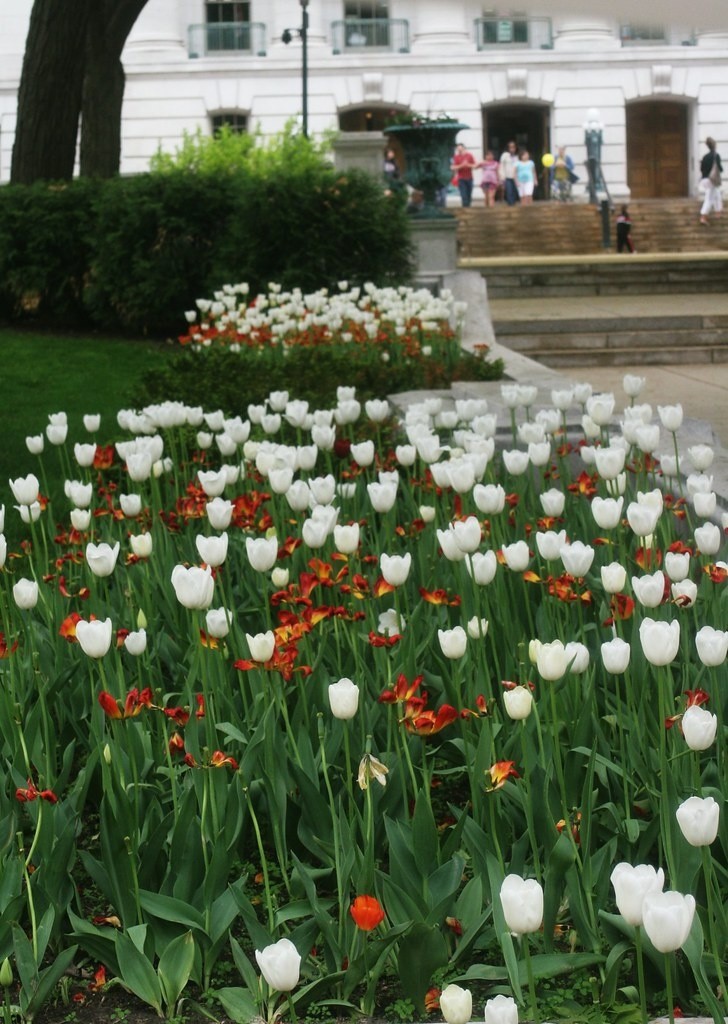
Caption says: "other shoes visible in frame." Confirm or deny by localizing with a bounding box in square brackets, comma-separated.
[715, 212, 727, 218]
[700, 215, 710, 226]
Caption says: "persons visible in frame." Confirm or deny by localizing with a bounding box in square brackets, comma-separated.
[450, 140, 579, 207]
[383, 148, 400, 193]
[616, 204, 636, 253]
[699, 137, 723, 226]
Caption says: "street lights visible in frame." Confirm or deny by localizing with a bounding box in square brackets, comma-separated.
[282, 27, 308, 137]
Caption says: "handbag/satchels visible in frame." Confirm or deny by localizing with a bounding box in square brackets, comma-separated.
[709, 153, 722, 187]
